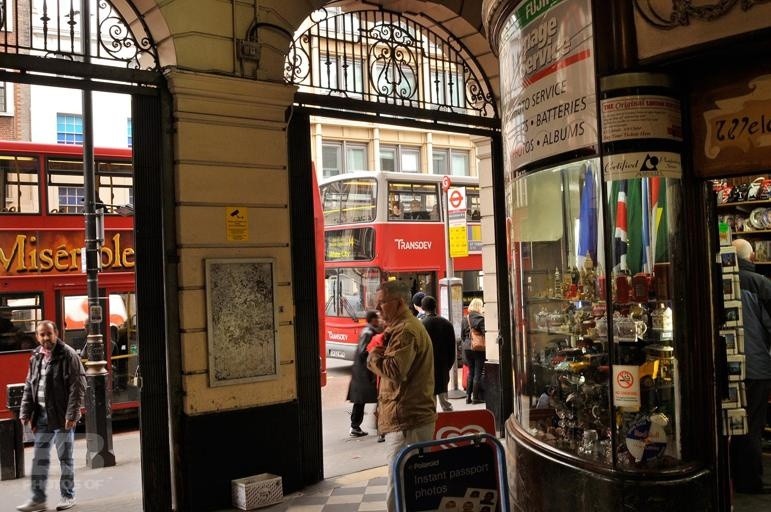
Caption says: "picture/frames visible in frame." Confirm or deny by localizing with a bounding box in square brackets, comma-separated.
[204, 257, 278, 388]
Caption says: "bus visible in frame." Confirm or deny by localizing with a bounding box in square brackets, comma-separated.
[0, 137, 138, 431]
[322, 167, 478, 367]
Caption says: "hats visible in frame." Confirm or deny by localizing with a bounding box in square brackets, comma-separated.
[413, 292, 425, 306]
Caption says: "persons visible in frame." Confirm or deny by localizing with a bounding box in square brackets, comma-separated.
[481, 491, 495, 505]
[346, 310, 384, 437]
[389, 202, 405, 218]
[420, 295, 457, 411]
[429, 203, 440, 220]
[366, 278, 439, 512]
[729, 238, 771, 493]
[462, 501, 475, 512]
[18, 335, 36, 349]
[461, 297, 485, 404]
[365, 325, 392, 443]
[0, 304, 23, 350]
[412, 291, 427, 320]
[404, 200, 431, 220]
[14, 319, 90, 512]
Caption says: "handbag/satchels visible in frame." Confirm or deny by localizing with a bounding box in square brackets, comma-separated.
[470, 329, 485, 352]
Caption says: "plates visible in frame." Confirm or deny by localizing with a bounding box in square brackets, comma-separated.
[750, 208, 771, 231]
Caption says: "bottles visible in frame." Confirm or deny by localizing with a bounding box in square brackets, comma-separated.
[533, 250, 649, 341]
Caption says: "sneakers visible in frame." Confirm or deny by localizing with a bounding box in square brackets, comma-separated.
[350, 429, 368, 436]
[17, 500, 46, 512]
[56, 495, 75, 510]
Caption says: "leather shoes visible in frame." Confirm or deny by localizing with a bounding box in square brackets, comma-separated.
[466, 398, 471, 404]
[472, 399, 483, 404]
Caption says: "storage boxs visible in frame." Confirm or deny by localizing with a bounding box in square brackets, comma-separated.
[230, 473, 283, 510]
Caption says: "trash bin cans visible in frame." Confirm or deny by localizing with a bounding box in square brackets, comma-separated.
[1, 418, 25, 480]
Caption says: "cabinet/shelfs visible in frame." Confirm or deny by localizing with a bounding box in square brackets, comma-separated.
[700, 165, 771, 277]
